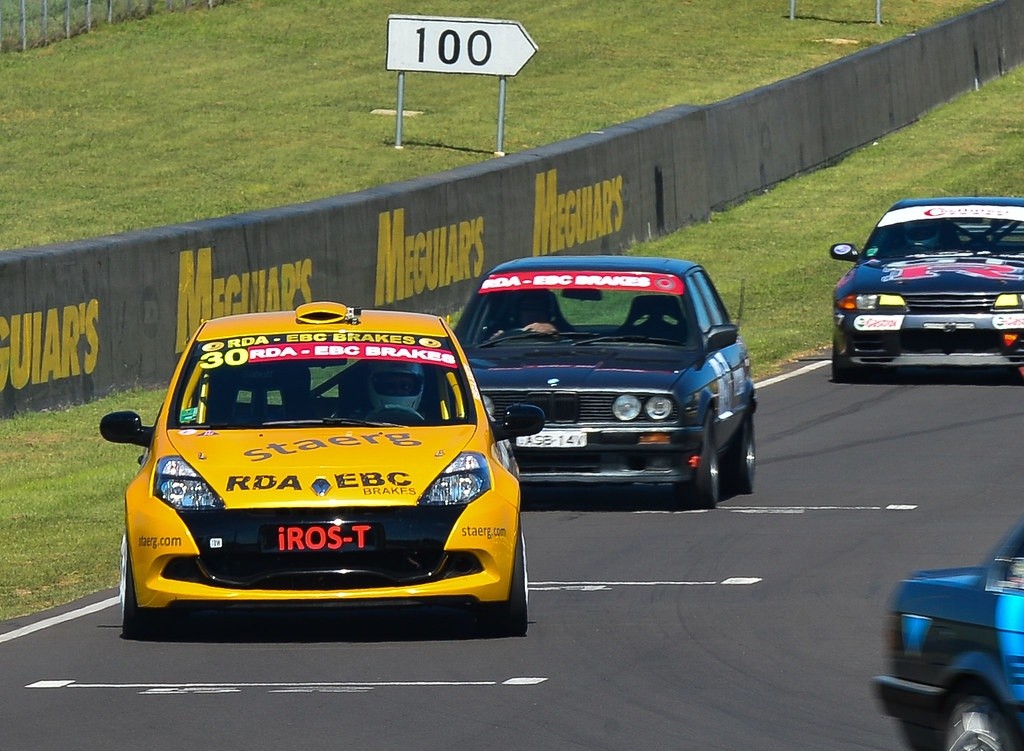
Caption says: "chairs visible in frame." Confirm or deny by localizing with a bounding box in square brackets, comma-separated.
[614, 295, 686, 343]
[478, 290, 576, 343]
[205, 363, 311, 426]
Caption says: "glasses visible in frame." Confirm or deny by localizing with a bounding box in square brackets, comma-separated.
[382, 383, 415, 393]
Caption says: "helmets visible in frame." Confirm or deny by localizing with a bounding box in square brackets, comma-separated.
[367, 360, 424, 410]
[904, 221, 947, 251]
[516, 292, 555, 324]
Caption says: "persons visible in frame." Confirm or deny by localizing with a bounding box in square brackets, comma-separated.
[489, 290, 557, 340]
[903, 220, 940, 249]
[352, 359, 428, 422]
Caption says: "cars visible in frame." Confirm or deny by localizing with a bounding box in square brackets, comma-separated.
[865, 516, 1024, 751]
[96, 300, 548, 642]
[829, 196, 1024, 391]
[437, 256, 760, 510]
